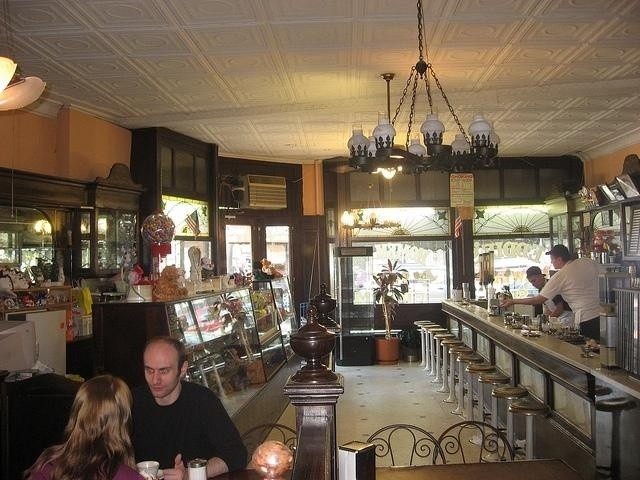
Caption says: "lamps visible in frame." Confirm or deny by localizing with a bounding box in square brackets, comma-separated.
[341, 181, 401, 230]
[348, 0, 501, 175]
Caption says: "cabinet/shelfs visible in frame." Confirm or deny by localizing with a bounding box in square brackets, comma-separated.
[72, 186, 142, 277]
[0, 286, 73, 340]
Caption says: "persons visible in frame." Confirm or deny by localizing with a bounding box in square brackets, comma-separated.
[526, 266, 572, 325]
[20, 375, 146, 480]
[125, 337, 249, 480]
[499, 244, 607, 341]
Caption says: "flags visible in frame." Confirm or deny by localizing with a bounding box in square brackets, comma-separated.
[185, 210, 201, 240]
[454, 216, 463, 238]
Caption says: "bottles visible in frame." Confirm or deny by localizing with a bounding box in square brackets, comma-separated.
[187, 458, 207, 480]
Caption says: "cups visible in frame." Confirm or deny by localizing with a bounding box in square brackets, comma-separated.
[504, 311, 599, 362]
[135, 461, 160, 480]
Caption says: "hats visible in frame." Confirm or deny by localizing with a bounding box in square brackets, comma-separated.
[527, 266, 547, 277]
[546, 245, 569, 256]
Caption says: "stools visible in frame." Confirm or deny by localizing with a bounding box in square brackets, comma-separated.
[414, 319, 551, 461]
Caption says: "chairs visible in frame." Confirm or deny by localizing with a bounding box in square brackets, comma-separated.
[367, 424, 445, 467]
[432, 421, 515, 463]
[240, 423, 298, 470]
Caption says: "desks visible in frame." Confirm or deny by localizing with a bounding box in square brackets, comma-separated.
[161, 457, 585, 480]
